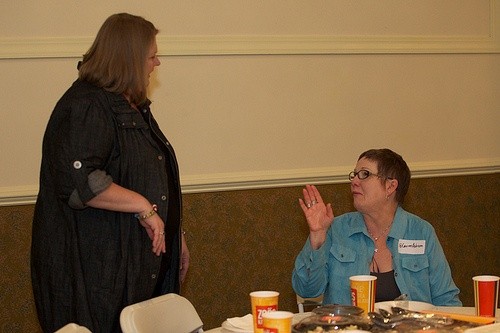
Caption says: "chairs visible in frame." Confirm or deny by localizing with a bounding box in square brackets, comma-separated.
[119, 292, 205, 333]
[296, 293, 323, 314]
[55, 322, 92, 333]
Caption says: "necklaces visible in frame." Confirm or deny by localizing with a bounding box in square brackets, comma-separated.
[368, 222, 391, 253]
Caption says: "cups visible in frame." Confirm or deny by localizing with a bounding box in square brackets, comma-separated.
[262, 311, 294, 333]
[250, 290, 280, 333]
[349, 275, 377, 313]
[472, 275, 500, 324]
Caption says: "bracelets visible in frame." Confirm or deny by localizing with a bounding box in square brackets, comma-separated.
[181, 230, 186, 235]
[135, 204, 157, 220]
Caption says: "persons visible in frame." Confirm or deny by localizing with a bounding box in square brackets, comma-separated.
[292, 149, 463, 307]
[30, 13, 189, 333]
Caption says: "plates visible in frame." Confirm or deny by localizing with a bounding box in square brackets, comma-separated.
[419, 309, 496, 333]
[465, 322, 500, 333]
[313, 305, 364, 314]
[375, 300, 437, 316]
[221, 316, 254, 333]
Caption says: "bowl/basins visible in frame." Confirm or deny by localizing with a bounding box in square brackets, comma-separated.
[291, 313, 373, 333]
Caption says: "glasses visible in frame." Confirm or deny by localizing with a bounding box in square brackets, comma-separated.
[348, 169, 398, 192]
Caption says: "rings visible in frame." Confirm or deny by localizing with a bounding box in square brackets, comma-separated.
[307, 203, 312, 209]
[311, 199, 317, 205]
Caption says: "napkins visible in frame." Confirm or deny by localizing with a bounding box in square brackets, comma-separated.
[222, 312, 255, 333]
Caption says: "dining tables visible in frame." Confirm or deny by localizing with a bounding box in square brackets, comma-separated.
[203, 305, 500, 333]
